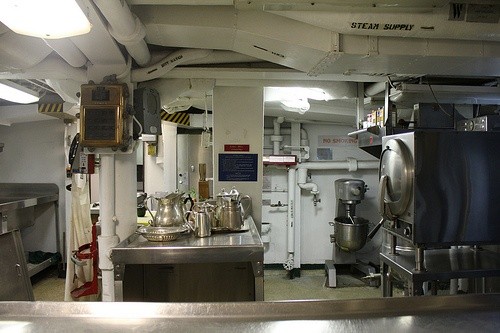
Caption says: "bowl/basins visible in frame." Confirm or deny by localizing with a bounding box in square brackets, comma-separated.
[136, 228, 186, 241]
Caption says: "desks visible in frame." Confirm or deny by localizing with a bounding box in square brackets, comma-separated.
[111, 215, 265, 303]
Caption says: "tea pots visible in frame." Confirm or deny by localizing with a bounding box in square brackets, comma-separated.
[145, 186, 252, 238]
